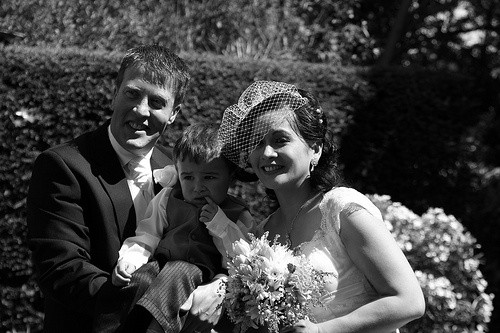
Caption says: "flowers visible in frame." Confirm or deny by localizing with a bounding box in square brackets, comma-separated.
[215, 231, 339, 333]
[153, 164, 177, 188]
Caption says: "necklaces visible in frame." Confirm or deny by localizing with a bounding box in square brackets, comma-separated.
[285, 205, 305, 247]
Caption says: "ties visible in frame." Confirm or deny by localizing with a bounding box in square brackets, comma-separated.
[128, 155, 152, 207]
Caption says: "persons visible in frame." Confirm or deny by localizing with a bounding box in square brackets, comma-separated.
[27, 46, 230, 333]
[216, 81, 425, 333]
[112, 123, 256, 333]
